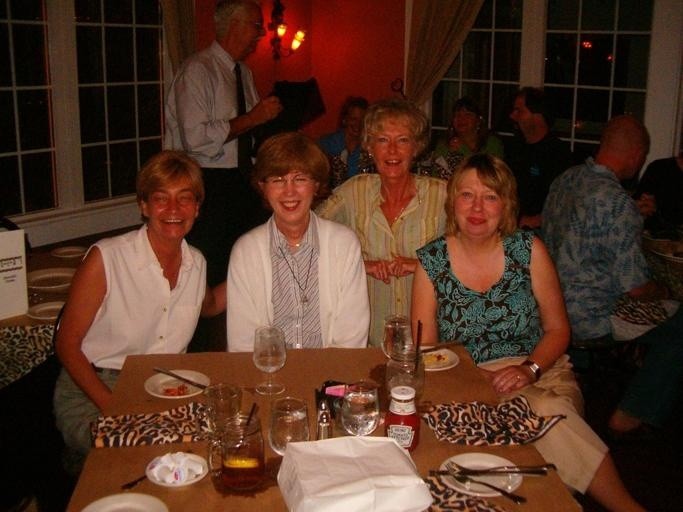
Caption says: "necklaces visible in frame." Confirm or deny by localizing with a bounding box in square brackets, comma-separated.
[277, 246, 315, 304]
[388, 206, 405, 227]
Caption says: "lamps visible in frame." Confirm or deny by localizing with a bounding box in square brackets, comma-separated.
[265, 2, 309, 66]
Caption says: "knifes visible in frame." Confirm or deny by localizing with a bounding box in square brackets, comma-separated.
[421, 340, 462, 352]
[153, 367, 207, 391]
[427, 468, 549, 477]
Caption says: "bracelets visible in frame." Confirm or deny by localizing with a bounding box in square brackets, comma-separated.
[521, 359, 541, 382]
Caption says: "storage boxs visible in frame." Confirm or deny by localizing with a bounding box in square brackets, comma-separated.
[0, 222, 29, 319]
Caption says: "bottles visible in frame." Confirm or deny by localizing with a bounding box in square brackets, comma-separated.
[384, 384, 419, 453]
[315, 398, 332, 443]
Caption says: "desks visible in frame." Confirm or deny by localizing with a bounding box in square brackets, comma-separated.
[0, 225, 150, 382]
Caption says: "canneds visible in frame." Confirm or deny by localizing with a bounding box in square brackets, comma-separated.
[222, 415, 265, 492]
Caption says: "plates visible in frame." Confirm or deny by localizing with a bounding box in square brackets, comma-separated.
[25, 301, 62, 320]
[145, 451, 208, 489]
[79, 492, 169, 512]
[646, 239, 683, 264]
[145, 368, 211, 400]
[437, 451, 524, 498]
[26, 267, 77, 290]
[49, 245, 89, 259]
[418, 344, 459, 372]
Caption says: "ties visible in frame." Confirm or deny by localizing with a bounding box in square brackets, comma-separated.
[234, 64, 253, 167]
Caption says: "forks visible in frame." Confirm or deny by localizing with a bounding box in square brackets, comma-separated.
[446, 459, 527, 508]
[448, 458, 557, 476]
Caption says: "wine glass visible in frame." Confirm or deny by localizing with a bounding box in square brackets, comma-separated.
[384, 358, 424, 398]
[249, 326, 288, 398]
[381, 316, 417, 385]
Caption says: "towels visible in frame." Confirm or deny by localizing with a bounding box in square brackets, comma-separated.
[420, 469, 503, 511]
[606, 292, 669, 327]
[426, 391, 567, 448]
[86, 403, 216, 445]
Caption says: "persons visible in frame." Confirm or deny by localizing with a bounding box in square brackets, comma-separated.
[637, 150, 682, 239]
[314, 96, 374, 181]
[541, 114, 668, 439]
[409, 152, 649, 509]
[158, 1, 283, 250]
[503, 84, 572, 233]
[311, 95, 451, 348]
[224, 129, 374, 351]
[420, 97, 516, 182]
[49, 148, 227, 471]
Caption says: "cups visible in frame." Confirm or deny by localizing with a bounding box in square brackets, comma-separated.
[202, 381, 241, 442]
[206, 413, 263, 496]
[340, 379, 377, 440]
[267, 395, 310, 457]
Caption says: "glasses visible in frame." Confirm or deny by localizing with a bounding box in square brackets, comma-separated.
[263, 174, 316, 188]
